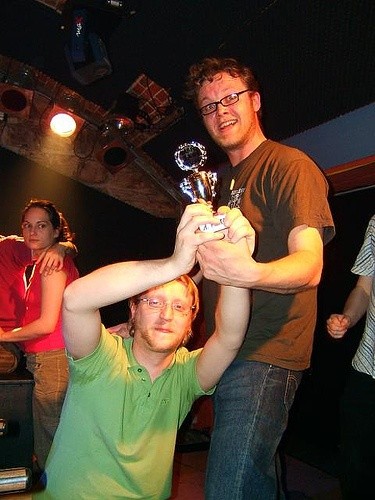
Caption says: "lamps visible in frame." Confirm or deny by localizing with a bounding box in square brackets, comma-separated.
[40, 91, 87, 145]
[95, 115, 137, 176]
[1, 66, 37, 120]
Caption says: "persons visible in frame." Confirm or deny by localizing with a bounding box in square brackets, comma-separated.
[31, 203, 258, 500]
[0, 199, 80, 478]
[326, 214, 375, 500]
[0, 234, 79, 372]
[188, 56, 334, 500]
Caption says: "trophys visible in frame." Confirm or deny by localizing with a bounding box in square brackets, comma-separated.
[174, 141, 230, 234]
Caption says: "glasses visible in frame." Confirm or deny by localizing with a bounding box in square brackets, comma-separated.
[135, 297, 195, 315]
[196, 88, 255, 116]
